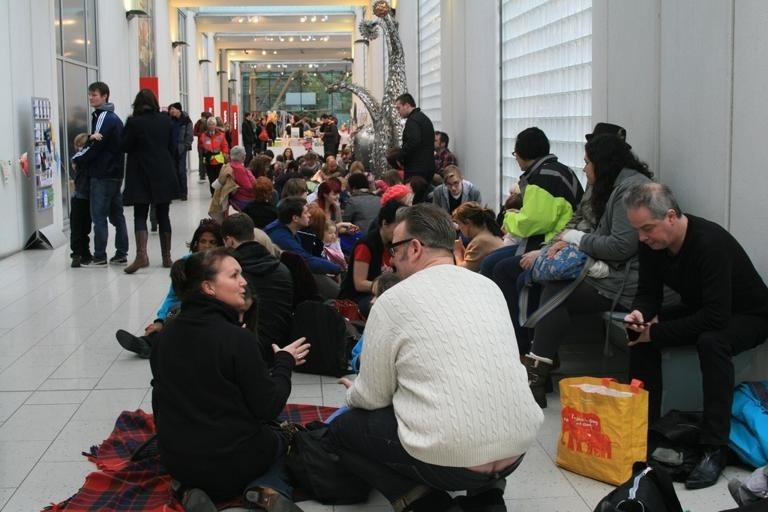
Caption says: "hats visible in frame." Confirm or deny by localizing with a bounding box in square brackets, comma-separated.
[585, 123, 632, 150]
[380, 184, 413, 205]
[515, 127, 549, 160]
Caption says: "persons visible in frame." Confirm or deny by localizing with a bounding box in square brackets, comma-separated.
[67, 83, 231, 273]
[623, 182, 768, 489]
[396, 93, 436, 205]
[116, 122, 681, 408]
[149, 250, 310, 511]
[328, 204, 544, 512]
[241, 110, 352, 168]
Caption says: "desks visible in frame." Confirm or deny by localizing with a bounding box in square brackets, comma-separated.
[266, 137, 348, 165]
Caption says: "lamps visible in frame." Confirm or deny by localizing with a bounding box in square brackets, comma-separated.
[126, 9, 236, 83]
[342, 39, 369, 62]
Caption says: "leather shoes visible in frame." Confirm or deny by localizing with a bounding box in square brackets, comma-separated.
[115, 330, 151, 359]
[685, 446, 726, 490]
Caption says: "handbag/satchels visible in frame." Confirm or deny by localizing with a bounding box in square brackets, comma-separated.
[283, 423, 373, 505]
[524, 243, 588, 286]
[555, 376, 650, 487]
[293, 300, 356, 378]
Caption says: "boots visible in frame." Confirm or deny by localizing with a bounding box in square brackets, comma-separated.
[160, 232, 173, 266]
[124, 230, 149, 274]
[522, 354, 553, 409]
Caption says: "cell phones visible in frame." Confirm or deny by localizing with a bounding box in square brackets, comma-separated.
[610, 316, 648, 326]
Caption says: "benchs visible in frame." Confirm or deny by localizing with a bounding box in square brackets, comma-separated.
[602, 312, 750, 420]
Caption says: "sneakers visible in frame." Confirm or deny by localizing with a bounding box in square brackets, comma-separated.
[80, 259, 107, 268]
[109, 256, 127, 266]
[71, 255, 81, 267]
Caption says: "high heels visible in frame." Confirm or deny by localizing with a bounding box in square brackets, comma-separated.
[246, 486, 303, 512]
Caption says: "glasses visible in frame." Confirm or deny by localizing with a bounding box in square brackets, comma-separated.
[386, 238, 424, 257]
[512, 151, 517, 156]
